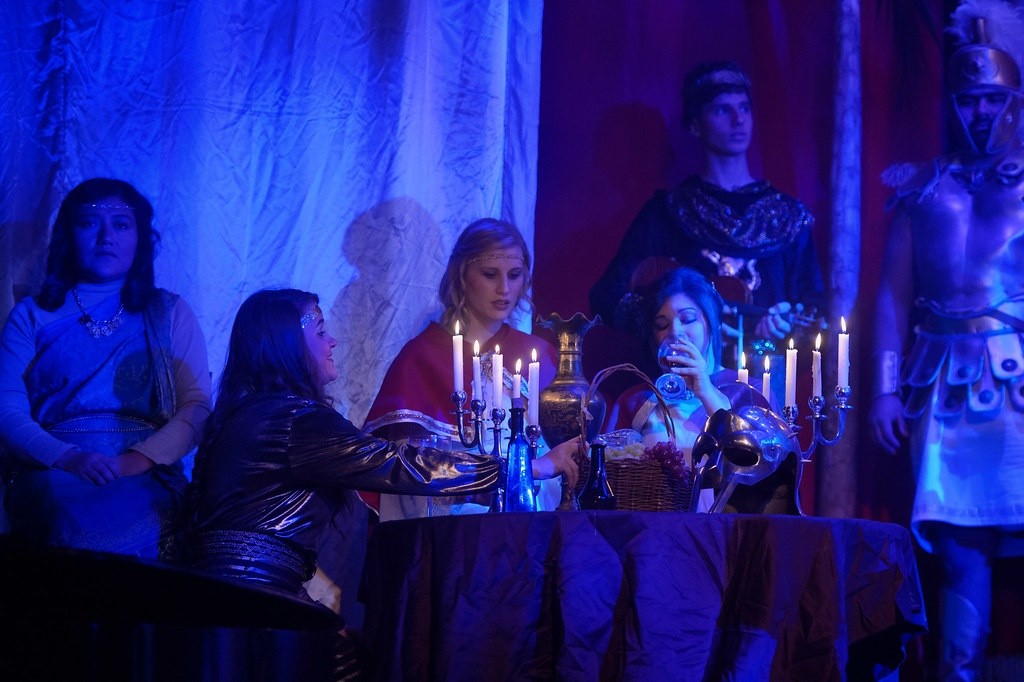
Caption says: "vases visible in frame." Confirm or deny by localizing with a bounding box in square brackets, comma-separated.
[536, 312, 606, 511]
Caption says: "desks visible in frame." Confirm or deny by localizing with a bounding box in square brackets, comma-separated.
[347, 509, 930, 682]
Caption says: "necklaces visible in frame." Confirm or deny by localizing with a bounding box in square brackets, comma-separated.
[71, 289, 126, 339]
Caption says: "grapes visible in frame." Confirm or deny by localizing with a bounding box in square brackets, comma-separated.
[606, 440, 689, 489]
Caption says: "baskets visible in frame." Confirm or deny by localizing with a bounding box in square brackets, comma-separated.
[561, 363, 696, 514]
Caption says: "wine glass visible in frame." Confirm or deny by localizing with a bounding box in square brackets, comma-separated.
[654, 337, 690, 402]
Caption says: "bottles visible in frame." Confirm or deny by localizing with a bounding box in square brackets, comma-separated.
[504, 398, 538, 513]
[578, 444, 617, 510]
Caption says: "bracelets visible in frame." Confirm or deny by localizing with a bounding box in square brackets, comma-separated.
[871, 350, 903, 401]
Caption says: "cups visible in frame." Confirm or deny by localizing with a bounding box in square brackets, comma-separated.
[410, 435, 452, 516]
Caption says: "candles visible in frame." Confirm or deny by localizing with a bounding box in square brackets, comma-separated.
[452, 320, 464, 392]
[812, 333, 822, 397]
[528, 348, 539, 426]
[513, 359, 522, 399]
[491, 345, 502, 409]
[785, 338, 798, 406]
[473, 340, 482, 402]
[738, 352, 748, 384]
[837, 316, 849, 387]
[762, 356, 770, 403]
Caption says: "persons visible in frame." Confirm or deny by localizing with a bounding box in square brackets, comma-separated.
[0, 177, 213, 564]
[590, 60, 822, 409]
[599, 265, 774, 513]
[355, 218, 560, 523]
[184, 288, 590, 682]
[865, 0, 1024, 682]
